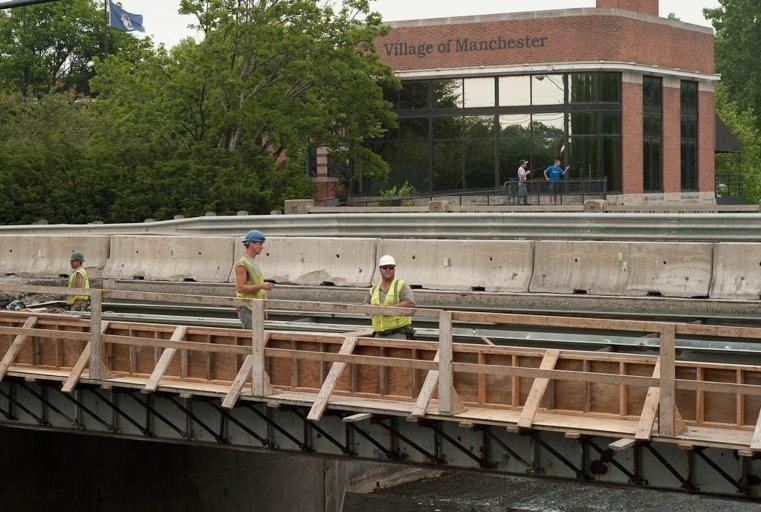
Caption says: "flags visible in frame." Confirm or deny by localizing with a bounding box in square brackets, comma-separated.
[108, 2, 148, 34]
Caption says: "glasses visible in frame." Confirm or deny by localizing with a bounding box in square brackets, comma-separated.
[382, 266, 394, 270]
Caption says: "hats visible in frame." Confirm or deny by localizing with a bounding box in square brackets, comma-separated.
[520, 160, 528, 164]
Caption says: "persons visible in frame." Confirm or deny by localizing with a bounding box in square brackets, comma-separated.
[543, 159, 570, 205]
[516, 159, 531, 205]
[366, 254, 416, 340]
[234, 229, 274, 329]
[65, 251, 89, 311]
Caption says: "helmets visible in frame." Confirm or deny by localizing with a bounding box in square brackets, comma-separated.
[242, 230, 265, 242]
[377, 254, 396, 267]
[69, 251, 84, 261]
